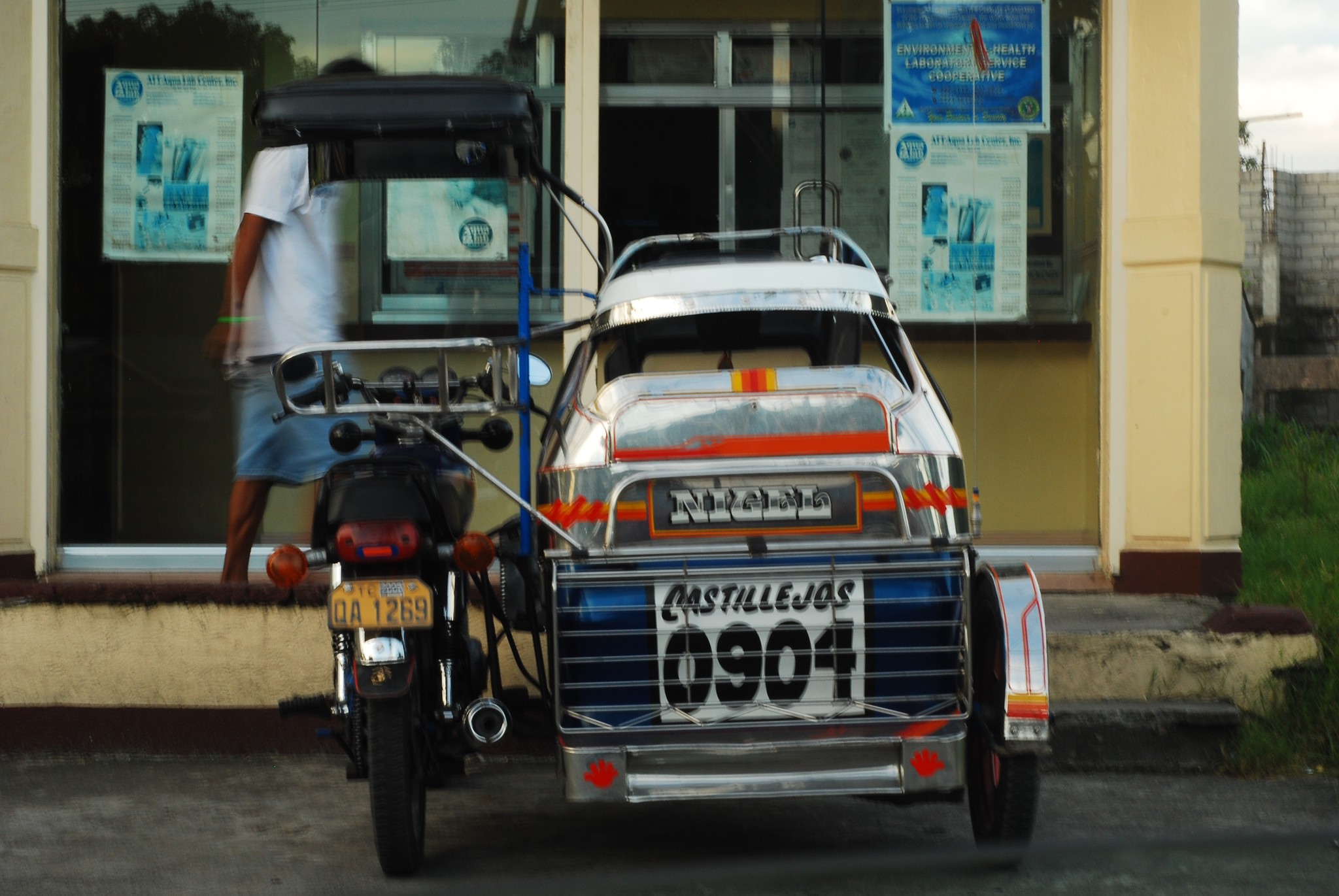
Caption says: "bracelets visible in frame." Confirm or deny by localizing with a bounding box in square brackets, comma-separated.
[219, 318, 253, 322]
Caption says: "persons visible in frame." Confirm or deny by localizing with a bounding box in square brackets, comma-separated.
[214, 57, 383, 591]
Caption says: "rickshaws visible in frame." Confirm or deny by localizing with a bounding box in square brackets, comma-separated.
[243, 63, 1060, 877]
